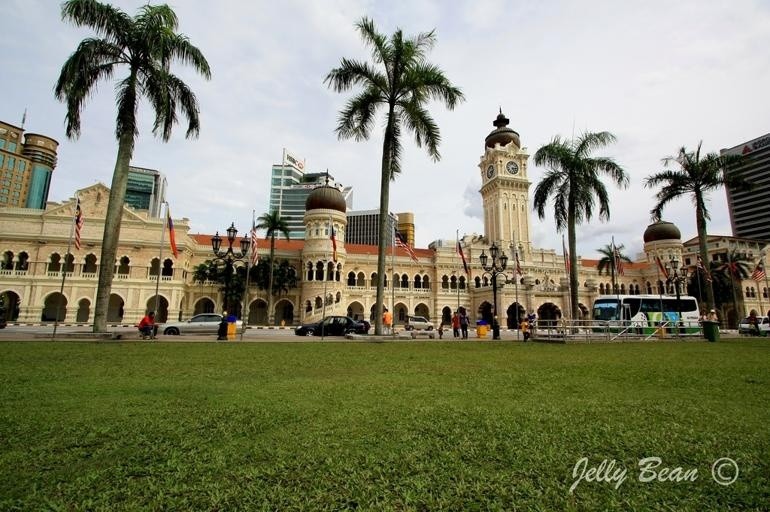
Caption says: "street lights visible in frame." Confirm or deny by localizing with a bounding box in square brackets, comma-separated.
[663, 254, 688, 339]
[212, 222, 252, 342]
[479, 241, 508, 340]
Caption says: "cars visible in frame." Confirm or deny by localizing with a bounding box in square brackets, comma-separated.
[157, 313, 247, 336]
[295, 315, 369, 337]
[405, 313, 436, 332]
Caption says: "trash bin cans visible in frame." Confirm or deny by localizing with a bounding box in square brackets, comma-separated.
[476, 320, 487, 339]
[702, 321, 720, 342]
[227, 315, 236, 339]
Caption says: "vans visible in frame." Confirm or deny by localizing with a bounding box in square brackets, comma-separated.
[738, 314, 770, 336]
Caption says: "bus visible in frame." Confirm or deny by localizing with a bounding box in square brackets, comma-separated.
[589, 293, 702, 337]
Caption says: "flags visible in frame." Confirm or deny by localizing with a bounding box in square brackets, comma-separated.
[697, 255, 714, 283]
[331, 226, 339, 263]
[456, 239, 471, 274]
[167, 207, 179, 260]
[517, 251, 522, 278]
[652, 251, 671, 281]
[395, 231, 420, 265]
[564, 243, 571, 275]
[75, 202, 85, 251]
[752, 260, 766, 282]
[250, 214, 260, 269]
[614, 248, 625, 276]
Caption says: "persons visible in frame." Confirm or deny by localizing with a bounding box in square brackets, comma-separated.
[698, 309, 708, 334]
[451, 312, 459, 337]
[437, 323, 444, 339]
[520, 317, 531, 342]
[458, 311, 470, 338]
[747, 310, 761, 337]
[138, 312, 159, 339]
[707, 309, 719, 322]
[748, 322, 756, 337]
[382, 309, 392, 335]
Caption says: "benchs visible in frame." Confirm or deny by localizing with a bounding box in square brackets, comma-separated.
[112, 328, 140, 339]
[411, 329, 437, 339]
